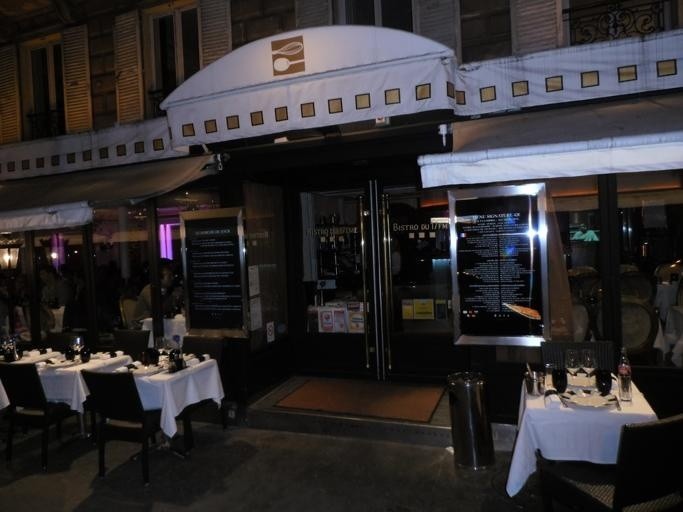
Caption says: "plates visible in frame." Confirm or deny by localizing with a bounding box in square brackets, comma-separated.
[559, 390, 618, 409]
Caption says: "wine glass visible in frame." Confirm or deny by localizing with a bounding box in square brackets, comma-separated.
[565, 349, 597, 391]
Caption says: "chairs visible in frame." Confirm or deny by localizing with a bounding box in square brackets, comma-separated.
[539, 339, 682, 511]
[1, 328, 224, 488]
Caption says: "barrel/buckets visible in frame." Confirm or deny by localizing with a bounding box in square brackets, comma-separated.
[524, 372, 545, 395]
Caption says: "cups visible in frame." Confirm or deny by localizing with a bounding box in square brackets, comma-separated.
[546, 363, 567, 392]
[596, 370, 612, 392]
[66, 349, 89, 364]
[175, 359, 183, 371]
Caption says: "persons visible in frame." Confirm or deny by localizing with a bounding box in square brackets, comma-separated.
[0, 244, 186, 343]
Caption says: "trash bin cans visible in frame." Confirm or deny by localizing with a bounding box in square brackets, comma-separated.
[446, 368, 497, 472]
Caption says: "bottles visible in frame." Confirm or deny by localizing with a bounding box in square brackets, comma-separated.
[169, 353, 175, 373]
[618, 349, 633, 402]
